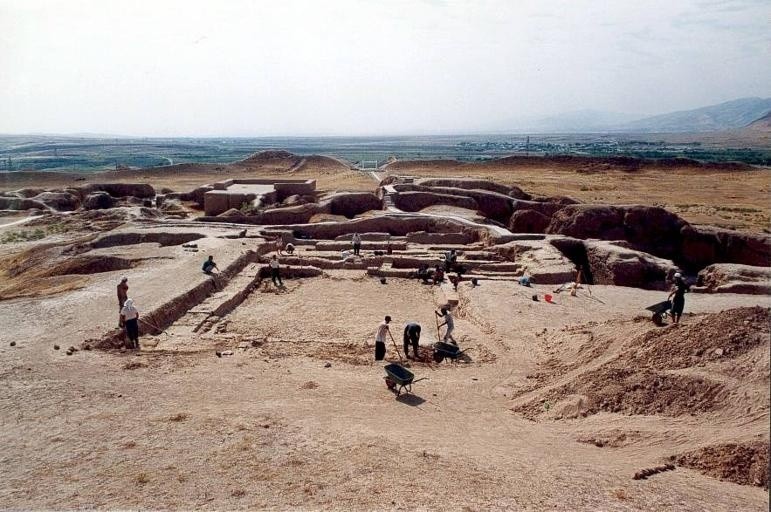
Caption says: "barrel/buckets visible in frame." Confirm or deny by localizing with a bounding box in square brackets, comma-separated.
[472, 278, 477, 285]
[526, 282, 530, 287]
[381, 279, 386, 284]
[532, 295, 538, 301]
[388, 248, 392, 255]
[545, 293, 552, 301]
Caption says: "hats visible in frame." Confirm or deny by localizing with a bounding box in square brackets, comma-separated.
[674, 273, 681, 278]
[384, 316, 393, 321]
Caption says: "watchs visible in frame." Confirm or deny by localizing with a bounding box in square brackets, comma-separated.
[375, 315, 393, 359]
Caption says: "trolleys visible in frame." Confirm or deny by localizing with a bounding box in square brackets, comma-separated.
[432, 341, 474, 364]
[646, 295, 673, 325]
[383, 362, 413, 398]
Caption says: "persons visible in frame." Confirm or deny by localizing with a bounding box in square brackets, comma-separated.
[519, 275, 532, 287]
[285, 242, 296, 254]
[120, 298, 141, 349]
[404, 322, 423, 359]
[668, 272, 686, 323]
[352, 232, 361, 256]
[202, 255, 223, 290]
[415, 248, 479, 289]
[274, 233, 284, 255]
[435, 306, 456, 344]
[117, 276, 130, 328]
[268, 254, 284, 286]
[239, 228, 248, 237]
